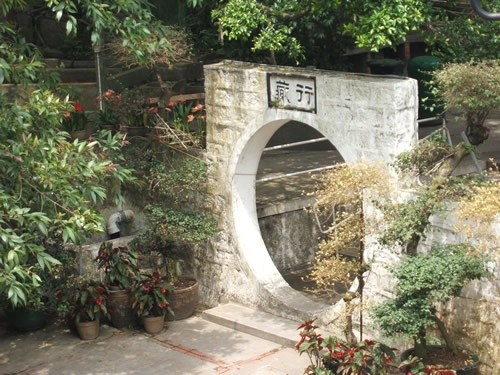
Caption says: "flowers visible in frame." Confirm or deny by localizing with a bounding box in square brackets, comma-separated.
[56, 242, 175, 320]
[295, 317, 458, 375]
[95, 87, 206, 149]
[63, 103, 94, 131]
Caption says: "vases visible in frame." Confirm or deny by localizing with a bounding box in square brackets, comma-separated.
[71, 131, 86, 140]
[75, 319, 100, 340]
[106, 289, 132, 325]
[141, 316, 165, 334]
[103, 124, 116, 134]
[128, 126, 147, 137]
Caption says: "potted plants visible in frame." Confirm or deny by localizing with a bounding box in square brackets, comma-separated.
[0, 73, 148, 332]
[128, 159, 226, 321]
[372, 132, 496, 375]
